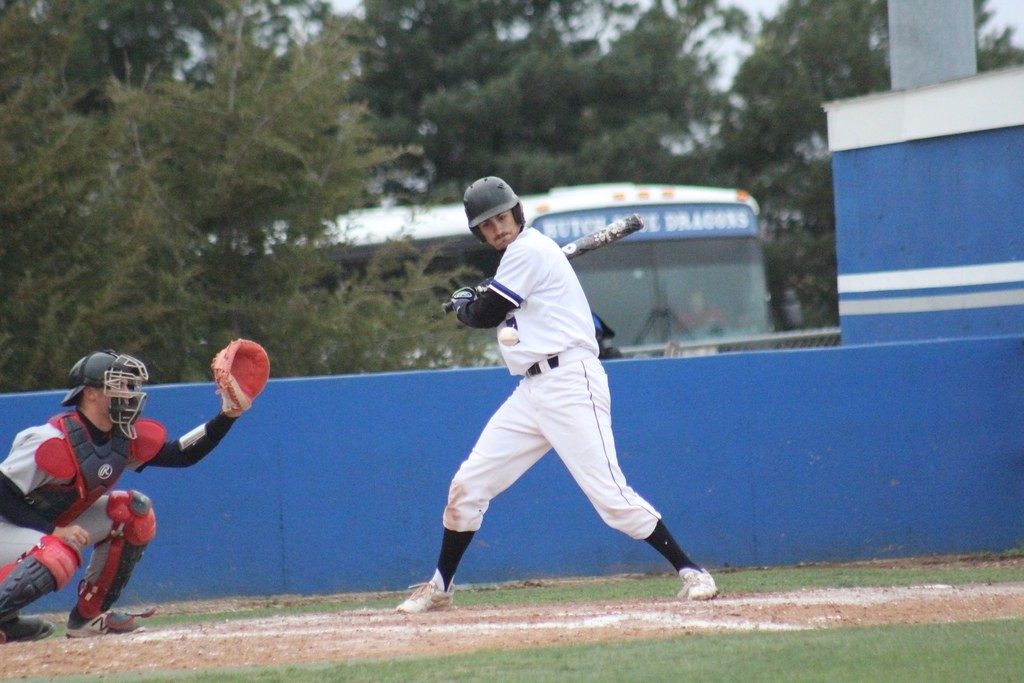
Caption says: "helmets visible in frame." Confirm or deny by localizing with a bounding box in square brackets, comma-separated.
[464, 176, 525, 242]
[62, 352, 120, 407]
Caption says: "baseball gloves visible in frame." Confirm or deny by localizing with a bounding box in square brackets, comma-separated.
[211, 337, 270, 417]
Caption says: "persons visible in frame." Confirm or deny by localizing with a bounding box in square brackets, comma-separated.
[675, 294, 726, 332]
[395, 175, 719, 613]
[0, 338, 271, 644]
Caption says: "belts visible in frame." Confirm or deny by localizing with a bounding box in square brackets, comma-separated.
[528, 356, 559, 377]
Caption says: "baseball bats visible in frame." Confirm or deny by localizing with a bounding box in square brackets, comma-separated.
[439, 213, 645, 315]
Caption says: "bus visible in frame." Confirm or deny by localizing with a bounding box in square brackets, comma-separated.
[198, 179, 804, 355]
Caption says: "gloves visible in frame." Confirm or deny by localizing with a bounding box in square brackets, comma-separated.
[450, 285, 478, 313]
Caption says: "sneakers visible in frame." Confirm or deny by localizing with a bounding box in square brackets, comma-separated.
[0, 618, 55, 643]
[678, 568, 718, 600]
[66, 607, 145, 636]
[395, 582, 454, 613]
